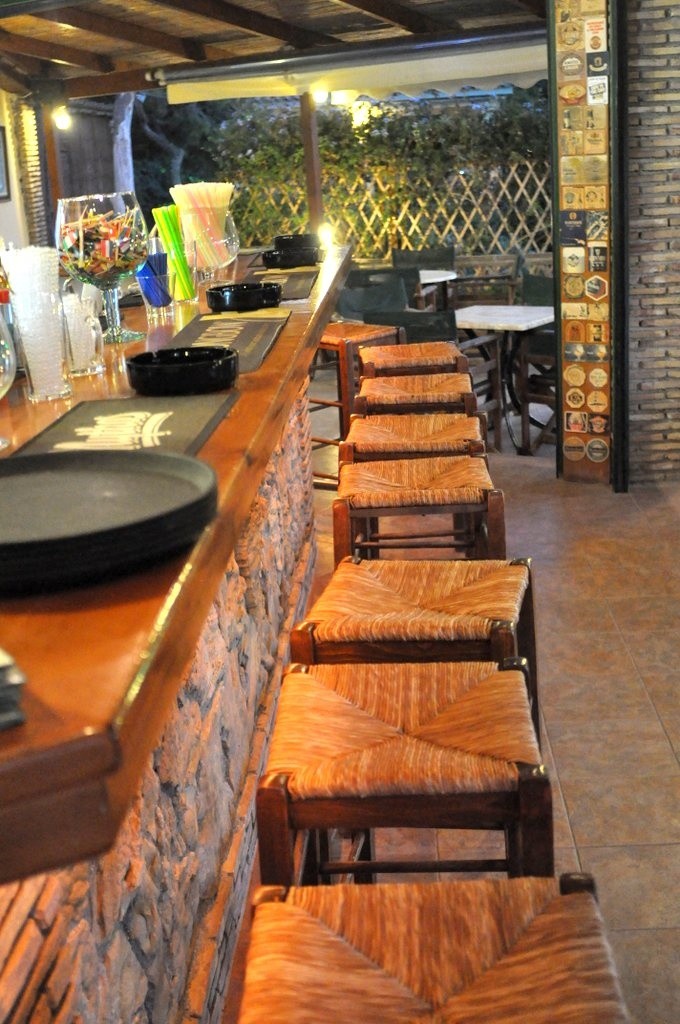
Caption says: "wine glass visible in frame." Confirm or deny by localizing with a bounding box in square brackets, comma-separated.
[55, 190, 148, 345]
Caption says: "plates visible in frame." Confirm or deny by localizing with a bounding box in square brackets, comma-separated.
[0, 450, 216, 586]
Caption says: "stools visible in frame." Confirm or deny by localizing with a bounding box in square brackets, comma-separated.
[254, 655, 556, 888]
[304, 322, 506, 558]
[289, 552, 543, 745]
[219, 875, 638, 1024]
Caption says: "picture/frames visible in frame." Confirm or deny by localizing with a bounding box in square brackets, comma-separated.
[0, 126, 11, 200]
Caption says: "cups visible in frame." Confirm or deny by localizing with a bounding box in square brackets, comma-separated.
[163, 241, 197, 305]
[62, 313, 108, 379]
[134, 272, 176, 317]
[183, 212, 238, 290]
[14, 310, 68, 400]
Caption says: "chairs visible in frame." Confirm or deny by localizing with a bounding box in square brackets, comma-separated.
[333, 243, 557, 451]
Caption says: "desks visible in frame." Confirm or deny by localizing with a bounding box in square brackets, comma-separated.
[416, 269, 457, 285]
[454, 303, 555, 432]
